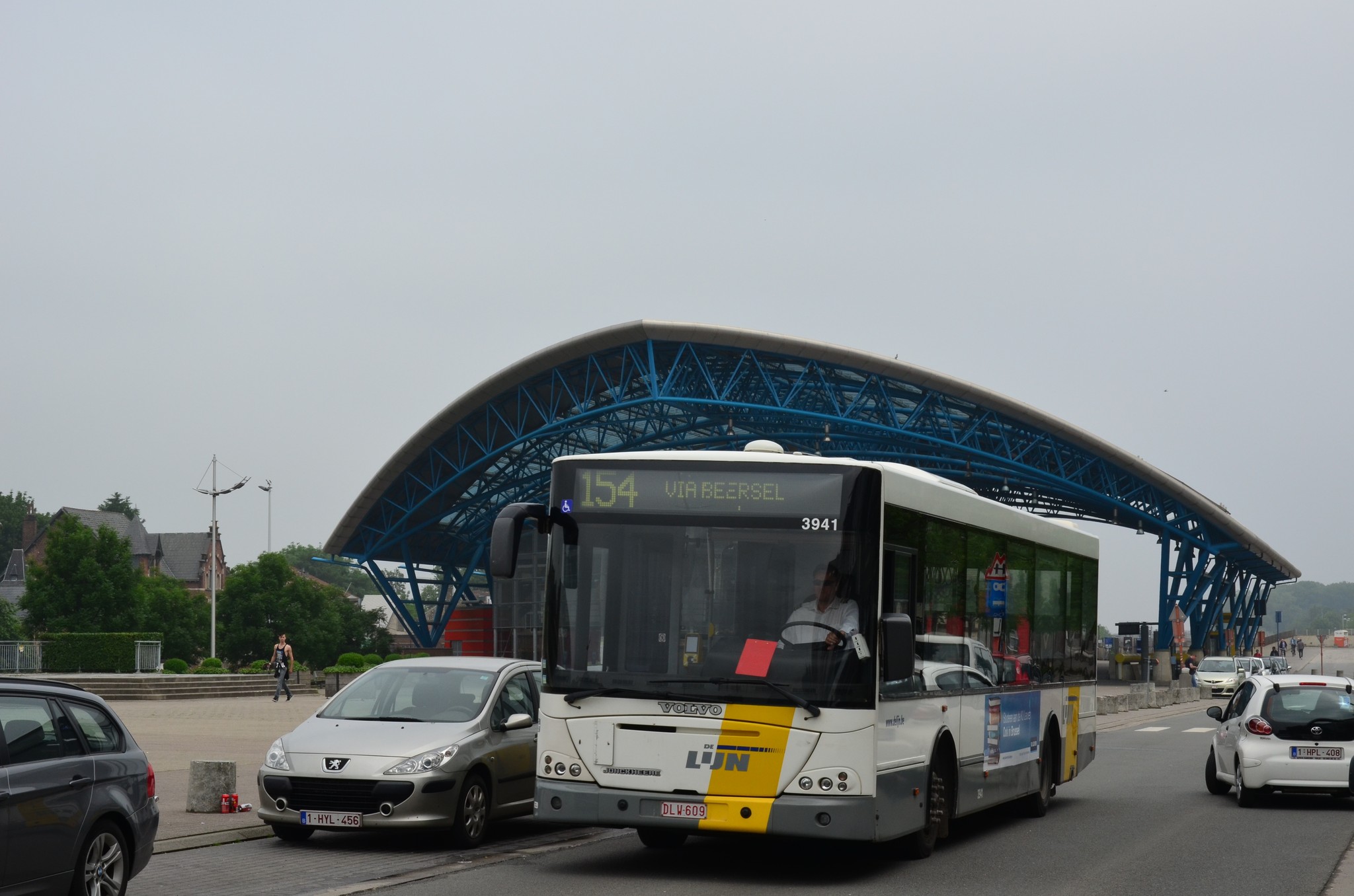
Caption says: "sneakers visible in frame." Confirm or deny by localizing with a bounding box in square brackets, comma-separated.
[286, 695, 293, 701]
[272, 696, 279, 702]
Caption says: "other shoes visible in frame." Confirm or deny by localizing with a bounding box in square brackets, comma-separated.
[1291, 651, 1292, 652]
[1295, 653, 1296, 655]
[1300, 657, 1302, 659]
[1284, 655, 1286, 657]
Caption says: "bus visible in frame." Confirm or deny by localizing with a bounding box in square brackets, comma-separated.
[488, 439, 1098, 865]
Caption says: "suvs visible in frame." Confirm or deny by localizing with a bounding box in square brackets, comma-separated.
[1, 676, 158, 896]
[1262, 657, 1282, 676]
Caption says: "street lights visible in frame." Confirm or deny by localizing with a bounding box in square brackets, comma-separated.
[1343, 614, 1352, 631]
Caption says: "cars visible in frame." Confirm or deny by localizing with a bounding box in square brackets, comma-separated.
[1268, 656, 1292, 674]
[1194, 655, 1245, 698]
[1273, 660, 1285, 675]
[1204, 675, 1353, 806]
[1237, 657, 1262, 678]
[1242, 656, 1271, 677]
[257, 655, 541, 842]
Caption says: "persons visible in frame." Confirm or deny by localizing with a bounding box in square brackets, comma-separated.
[774, 562, 859, 651]
[1289, 636, 1297, 655]
[1296, 638, 1300, 643]
[1270, 646, 1279, 657]
[266, 632, 295, 702]
[1279, 638, 1287, 657]
[1297, 639, 1305, 660]
[1184, 655, 1199, 688]
[1254, 650, 1262, 657]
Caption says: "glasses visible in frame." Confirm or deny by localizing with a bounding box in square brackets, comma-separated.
[813, 579, 836, 586]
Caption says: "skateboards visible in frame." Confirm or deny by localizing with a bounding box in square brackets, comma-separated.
[262, 662, 276, 671]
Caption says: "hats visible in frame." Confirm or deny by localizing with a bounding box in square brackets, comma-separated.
[1298, 638, 1300, 640]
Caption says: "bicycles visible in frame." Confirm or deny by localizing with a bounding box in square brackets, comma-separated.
[1290, 643, 1296, 657]
[1278, 648, 1286, 657]
